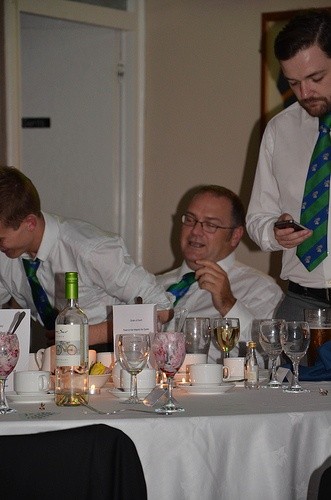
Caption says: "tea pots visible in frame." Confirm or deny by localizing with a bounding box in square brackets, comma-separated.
[36, 345, 55, 391]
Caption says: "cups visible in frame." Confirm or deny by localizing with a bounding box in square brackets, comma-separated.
[13, 371, 50, 395]
[224, 357, 244, 376]
[188, 364, 230, 386]
[175, 317, 211, 354]
[177, 352, 207, 373]
[89, 350, 115, 373]
[113, 360, 155, 392]
[304, 309, 331, 358]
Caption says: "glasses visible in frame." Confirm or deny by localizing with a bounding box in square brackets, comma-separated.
[181, 211, 235, 233]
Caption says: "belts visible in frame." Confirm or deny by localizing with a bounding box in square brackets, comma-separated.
[287, 281, 331, 303]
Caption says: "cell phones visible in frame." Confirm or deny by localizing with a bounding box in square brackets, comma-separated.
[275, 219, 307, 232]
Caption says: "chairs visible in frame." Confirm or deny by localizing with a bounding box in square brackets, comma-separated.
[0, 423, 148, 500]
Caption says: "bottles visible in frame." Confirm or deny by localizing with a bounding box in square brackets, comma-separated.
[244, 341, 258, 388]
[55, 272, 89, 406]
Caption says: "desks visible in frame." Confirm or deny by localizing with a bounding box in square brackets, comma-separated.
[0, 380, 331, 500]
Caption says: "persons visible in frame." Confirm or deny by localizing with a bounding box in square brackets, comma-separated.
[0, 166, 176, 353]
[155, 185, 284, 358]
[244, 10, 331, 381]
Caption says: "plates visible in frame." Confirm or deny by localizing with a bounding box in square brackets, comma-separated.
[177, 382, 234, 393]
[4, 391, 55, 411]
[222, 377, 244, 381]
[174, 374, 188, 383]
[108, 386, 151, 401]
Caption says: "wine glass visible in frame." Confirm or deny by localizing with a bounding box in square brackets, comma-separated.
[115, 334, 150, 403]
[258, 319, 288, 388]
[279, 321, 311, 393]
[0, 332, 20, 415]
[152, 331, 186, 413]
[212, 317, 240, 357]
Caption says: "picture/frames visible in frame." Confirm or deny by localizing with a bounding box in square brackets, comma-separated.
[261, 7, 329, 136]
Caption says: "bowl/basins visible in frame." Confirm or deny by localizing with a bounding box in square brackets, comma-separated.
[50, 373, 112, 388]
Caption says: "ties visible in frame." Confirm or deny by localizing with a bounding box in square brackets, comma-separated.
[296, 112, 331, 272]
[167, 273, 196, 305]
[23, 258, 55, 330]
